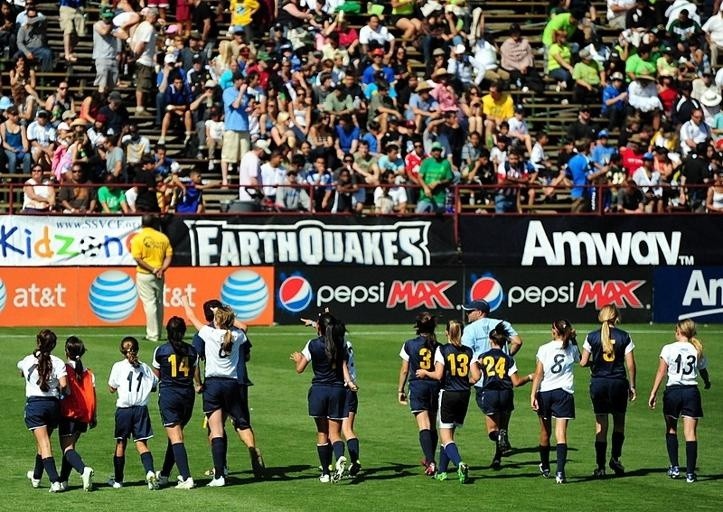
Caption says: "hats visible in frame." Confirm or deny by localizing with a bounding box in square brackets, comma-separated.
[598, 128, 654, 161]
[58, 110, 88, 131]
[164, 54, 177, 65]
[462, 299, 490, 311]
[165, 24, 179, 33]
[256, 139, 271, 155]
[367, 40, 484, 151]
[580, 50, 722, 107]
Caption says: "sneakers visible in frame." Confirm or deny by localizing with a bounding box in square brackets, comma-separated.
[422, 430, 508, 482]
[318, 457, 361, 483]
[539, 462, 551, 478]
[687, 473, 697, 483]
[667, 466, 680, 478]
[556, 472, 565, 484]
[27, 462, 263, 491]
[593, 469, 604, 477]
[609, 457, 623, 473]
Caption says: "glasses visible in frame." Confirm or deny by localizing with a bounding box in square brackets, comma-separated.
[343, 159, 352, 163]
[414, 145, 423, 148]
[288, 172, 296, 176]
[73, 170, 82, 173]
[33, 170, 42, 172]
[60, 87, 68, 89]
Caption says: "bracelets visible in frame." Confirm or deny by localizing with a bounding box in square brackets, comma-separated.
[398, 391, 405, 393]
[628, 384, 634, 390]
[310, 321, 314, 327]
[195, 380, 202, 386]
[152, 268, 157, 273]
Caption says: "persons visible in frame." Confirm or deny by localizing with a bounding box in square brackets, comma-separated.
[17, 330, 69, 494]
[397, 312, 443, 476]
[2, 2, 723, 217]
[108, 337, 160, 491]
[131, 215, 172, 341]
[415, 320, 482, 484]
[300, 317, 363, 479]
[59, 336, 97, 493]
[647, 318, 710, 484]
[180, 294, 265, 488]
[530, 319, 593, 484]
[190, 299, 264, 477]
[151, 316, 205, 490]
[459, 299, 522, 456]
[468, 321, 535, 471]
[288, 313, 347, 484]
[578, 304, 636, 479]
